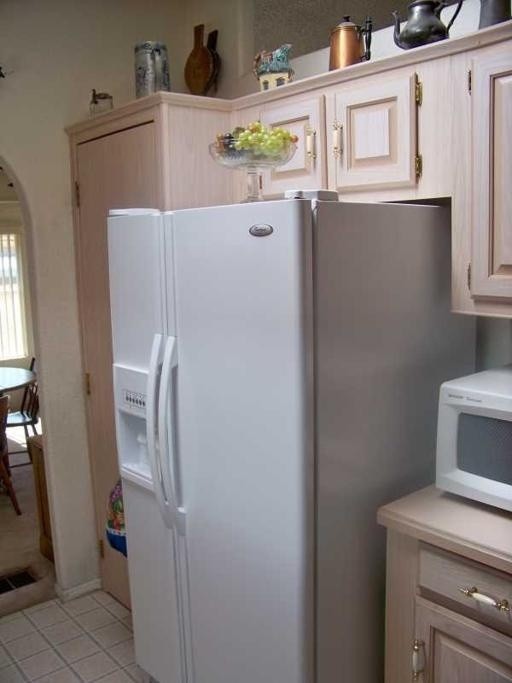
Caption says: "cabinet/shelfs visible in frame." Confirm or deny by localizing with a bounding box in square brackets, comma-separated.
[61, 91, 230, 609]
[379, 528, 512, 682]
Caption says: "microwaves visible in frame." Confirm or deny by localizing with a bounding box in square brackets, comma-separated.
[436, 363, 511, 514]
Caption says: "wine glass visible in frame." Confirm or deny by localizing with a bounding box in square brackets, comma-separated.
[209, 135, 296, 203]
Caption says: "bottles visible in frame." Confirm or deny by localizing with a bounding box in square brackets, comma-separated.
[89, 93, 115, 121]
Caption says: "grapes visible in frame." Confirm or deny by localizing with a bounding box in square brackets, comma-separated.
[214, 120, 299, 162]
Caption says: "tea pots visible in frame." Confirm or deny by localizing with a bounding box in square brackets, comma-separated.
[390, 0, 463, 51]
[327, 14, 373, 73]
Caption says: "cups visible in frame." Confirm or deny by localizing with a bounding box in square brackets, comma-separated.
[135, 40, 169, 98]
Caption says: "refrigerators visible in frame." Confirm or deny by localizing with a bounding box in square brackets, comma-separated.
[102, 188, 470, 681]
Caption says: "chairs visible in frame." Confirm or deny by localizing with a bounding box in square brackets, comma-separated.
[0, 355, 41, 515]
